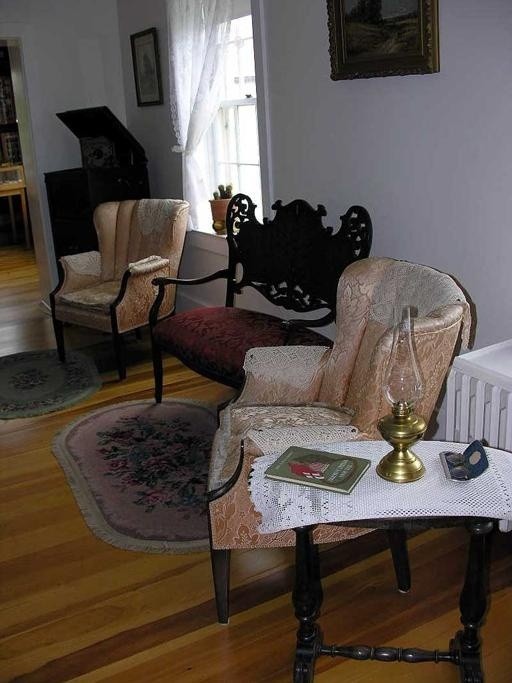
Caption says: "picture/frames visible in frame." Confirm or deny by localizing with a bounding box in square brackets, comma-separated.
[326, 1, 441, 81]
[130, 27, 165, 106]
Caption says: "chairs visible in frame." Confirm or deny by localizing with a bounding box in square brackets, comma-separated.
[203, 251, 472, 627]
[49, 196, 191, 384]
[149, 194, 374, 407]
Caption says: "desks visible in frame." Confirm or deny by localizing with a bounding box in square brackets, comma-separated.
[248, 438, 511, 682]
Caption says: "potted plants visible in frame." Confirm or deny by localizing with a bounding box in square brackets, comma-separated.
[208, 184, 236, 235]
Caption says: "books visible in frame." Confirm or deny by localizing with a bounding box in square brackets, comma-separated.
[263, 444, 371, 495]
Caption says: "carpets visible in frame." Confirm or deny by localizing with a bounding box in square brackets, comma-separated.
[1, 349, 103, 421]
[51, 397, 216, 556]
